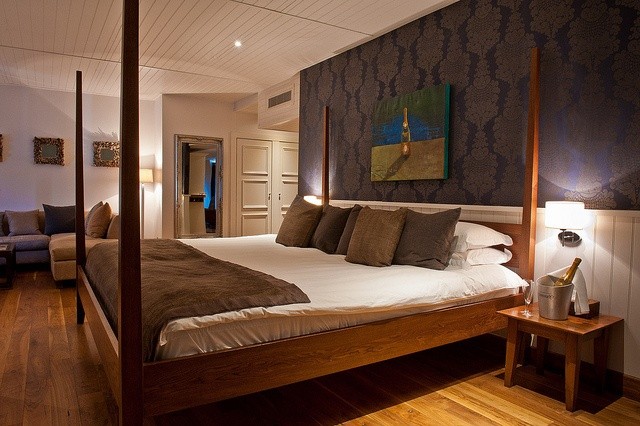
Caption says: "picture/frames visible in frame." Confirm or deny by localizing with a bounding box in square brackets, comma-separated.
[32, 136, 65, 166]
[370, 81, 451, 182]
[93, 141, 120, 167]
[0, 133, 9, 162]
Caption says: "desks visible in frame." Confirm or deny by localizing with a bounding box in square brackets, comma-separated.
[496, 301, 624, 412]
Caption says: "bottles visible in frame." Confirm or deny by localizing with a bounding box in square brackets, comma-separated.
[556, 258, 582, 285]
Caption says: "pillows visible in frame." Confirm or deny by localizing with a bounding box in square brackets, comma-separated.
[88, 202, 113, 237]
[42, 203, 76, 234]
[5, 209, 44, 236]
[85, 201, 103, 234]
[335, 204, 363, 254]
[448, 247, 513, 267]
[345, 205, 407, 267]
[274, 194, 324, 248]
[0, 214, 6, 236]
[311, 204, 354, 253]
[395, 205, 461, 268]
[453, 219, 513, 251]
[107, 215, 119, 238]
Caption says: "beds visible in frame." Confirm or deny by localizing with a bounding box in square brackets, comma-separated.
[75, 5, 541, 426]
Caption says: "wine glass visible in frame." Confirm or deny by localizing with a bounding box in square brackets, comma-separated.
[521, 281, 534, 316]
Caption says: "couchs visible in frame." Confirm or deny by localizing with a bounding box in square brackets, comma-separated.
[49, 226, 119, 282]
[0, 208, 50, 262]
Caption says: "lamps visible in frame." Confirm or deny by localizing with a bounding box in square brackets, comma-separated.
[544, 200, 588, 247]
